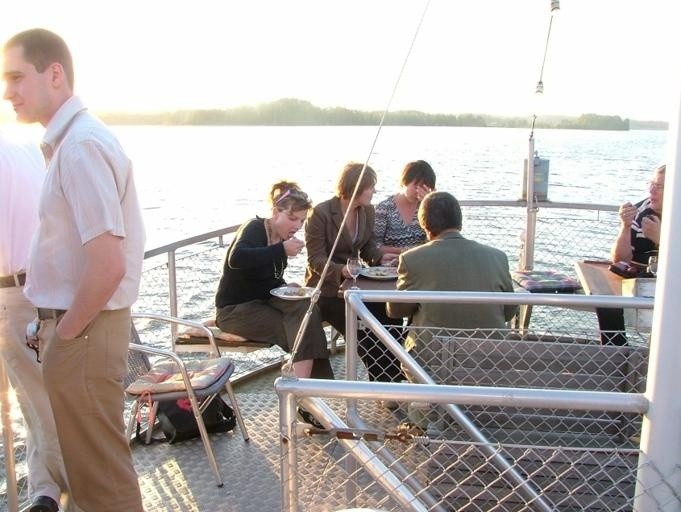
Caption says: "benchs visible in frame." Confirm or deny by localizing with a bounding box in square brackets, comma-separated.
[173, 315, 330, 358]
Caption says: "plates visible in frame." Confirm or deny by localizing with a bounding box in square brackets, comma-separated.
[359, 266, 398, 279]
[269, 286, 318, 301]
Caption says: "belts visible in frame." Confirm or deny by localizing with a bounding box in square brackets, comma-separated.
[36, 308, 67, 320]
[0, 273, 26, 288]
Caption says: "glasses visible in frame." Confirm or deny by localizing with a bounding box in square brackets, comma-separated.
[275, 189, 307, 204]
[646, 181, 663, 193]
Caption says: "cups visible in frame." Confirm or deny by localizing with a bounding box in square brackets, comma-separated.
[649, 255, 657, 277]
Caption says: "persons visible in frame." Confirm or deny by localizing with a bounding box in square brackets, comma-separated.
[593, 165, 666, 346]
[386, 192, 519, 385]
[303, 163, 407, 411]
[2, 29, 147, 511]
[0, 126, 79, 511]
[214, 179, 332, 434]
[372, 158, 437, 269]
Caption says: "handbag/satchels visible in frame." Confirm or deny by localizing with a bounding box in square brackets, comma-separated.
[151, 393, 236, 444]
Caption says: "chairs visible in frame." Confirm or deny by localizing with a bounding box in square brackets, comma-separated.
[124, 309, 249, 490]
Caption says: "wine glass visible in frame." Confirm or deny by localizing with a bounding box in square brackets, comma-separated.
[346, 258, 362, 290]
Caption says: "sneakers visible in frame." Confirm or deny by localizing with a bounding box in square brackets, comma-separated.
[27, 496, 59, 512]
[381, 400, 398, 408]
[296, 405, 325, 429]
